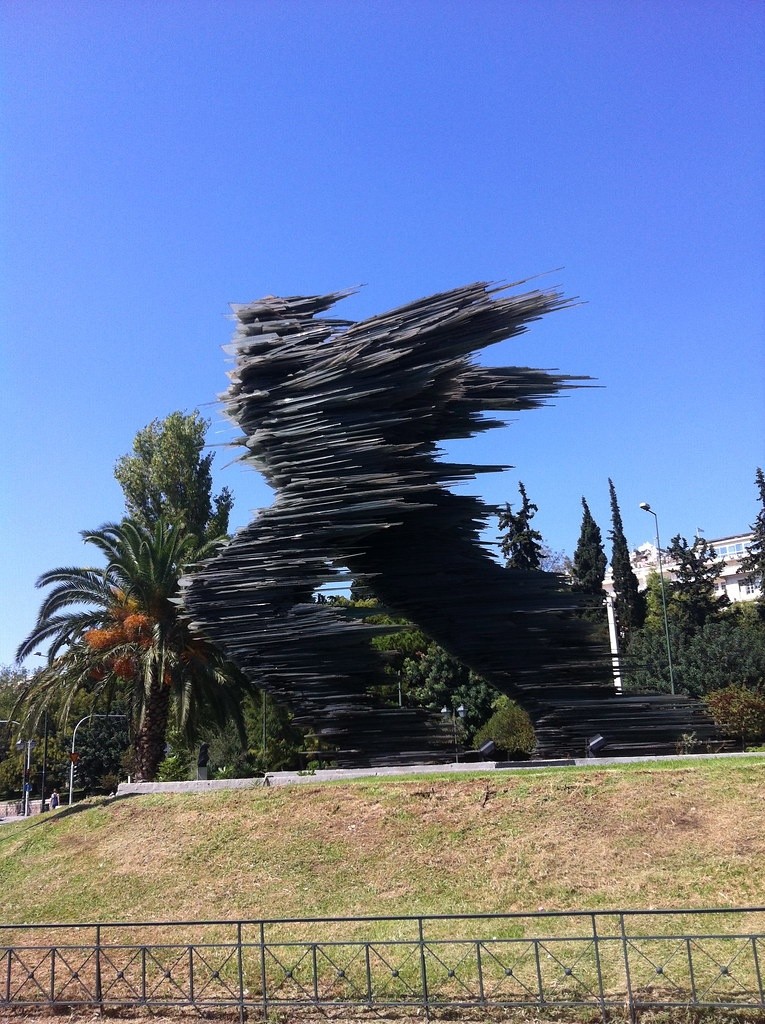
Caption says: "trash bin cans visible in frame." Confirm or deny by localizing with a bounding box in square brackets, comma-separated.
[40, 804, 49, 812]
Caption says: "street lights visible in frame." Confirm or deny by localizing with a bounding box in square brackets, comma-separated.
[440, 703, 469, 763]
[637, 500, 681, 743]
[32, 651, 53, 812]
[15, 738, 37, 817]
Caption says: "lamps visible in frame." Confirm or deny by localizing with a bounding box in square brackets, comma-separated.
[585, 733, 607, 759]
[480, 740, 497, 762]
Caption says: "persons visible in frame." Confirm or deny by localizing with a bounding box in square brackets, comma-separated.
[50, 788, 61, 810]
